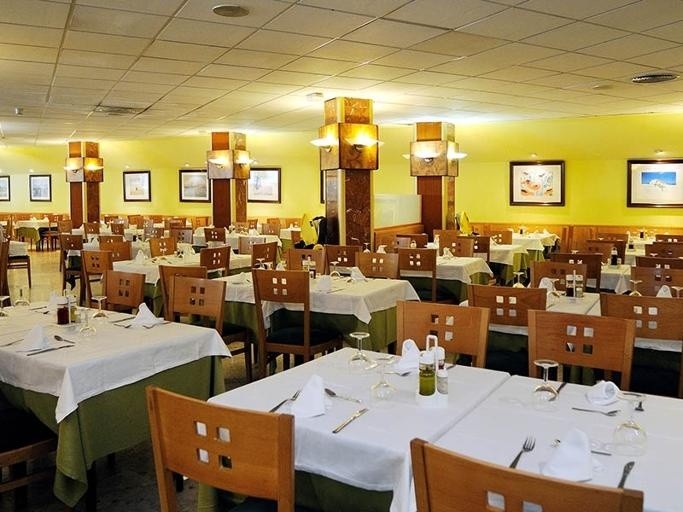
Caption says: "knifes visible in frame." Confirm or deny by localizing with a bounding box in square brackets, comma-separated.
[617, 460, 635, 488]
[332, 407, 368, 433]
[550, 381, 568, 401]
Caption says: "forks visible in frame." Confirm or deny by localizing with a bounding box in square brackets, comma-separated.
[268, 390, 300, 412]
[508, 434, 536, 469]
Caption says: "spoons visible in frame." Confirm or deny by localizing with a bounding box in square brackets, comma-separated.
[635, 401, 644, 412]
[571, 407, 622, 416]
[323, 388, 361, 403]
[384, 371, 411, 377]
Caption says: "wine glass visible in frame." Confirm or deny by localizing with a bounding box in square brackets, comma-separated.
[611, 391, 646, 457]
[370, 356, 393, 406]
[252, 226, 682, 299]
[347, 331, 370, 376]
[529, 358, 560, 410]
[0, 292, 111, 351]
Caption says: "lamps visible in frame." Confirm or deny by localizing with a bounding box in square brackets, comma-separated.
[346, 137, 377, 150]
[64, 166, 83, 172]
[206, 157, 228, 169]
[234, 155, 254, 167]
[311, 139, 336, 152]
[450, 151, 468, 162]
[84, 164, 104, 172]
[414, 151, 440, 163]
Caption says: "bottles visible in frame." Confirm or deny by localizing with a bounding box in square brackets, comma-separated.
[418, 345, 448, 396]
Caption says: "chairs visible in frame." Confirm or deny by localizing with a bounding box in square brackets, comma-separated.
[0, 214, 683, 512]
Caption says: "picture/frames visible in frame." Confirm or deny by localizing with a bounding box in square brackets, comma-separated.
[122, 170, 152, 201]
[0, 176, 10, 201]
[320, 170, 325, 203]
[627, 159, 683, 209]
[246, 167, 282, 203]
[178, 169, 211, 203]
[508, 159, 565, 207]
[29, 174, 51, 202]
